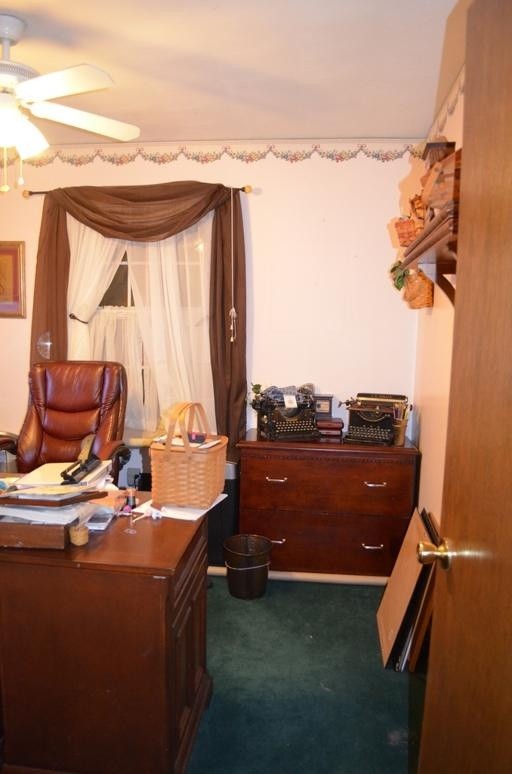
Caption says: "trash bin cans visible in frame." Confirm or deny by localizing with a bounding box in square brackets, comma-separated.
[222, 533, 272, 600]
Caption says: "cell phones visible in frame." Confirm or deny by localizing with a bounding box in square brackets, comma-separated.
[85, 510, 114, 532]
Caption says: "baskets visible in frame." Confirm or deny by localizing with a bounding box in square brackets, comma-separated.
[148, 402, 229, 510]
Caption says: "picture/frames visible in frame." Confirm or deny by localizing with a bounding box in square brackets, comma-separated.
[0, 238, 28, 319]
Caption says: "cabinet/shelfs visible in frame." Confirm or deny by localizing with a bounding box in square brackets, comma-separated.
[232, 427, 421, 580]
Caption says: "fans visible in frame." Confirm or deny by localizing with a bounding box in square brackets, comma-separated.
[0, 10, 140, 159]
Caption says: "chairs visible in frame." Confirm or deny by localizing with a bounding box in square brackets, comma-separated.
[1, 358, 128, 475]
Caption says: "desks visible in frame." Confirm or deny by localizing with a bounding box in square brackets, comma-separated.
[0, 469, 216, 773]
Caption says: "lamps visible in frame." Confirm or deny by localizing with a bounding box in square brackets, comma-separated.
[0, 90, 50, 194]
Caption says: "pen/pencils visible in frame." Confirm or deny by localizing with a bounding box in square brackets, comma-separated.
[392, 402, 411, 420]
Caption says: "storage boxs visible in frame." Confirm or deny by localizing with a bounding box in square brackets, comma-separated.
[0, 514, 79, 550]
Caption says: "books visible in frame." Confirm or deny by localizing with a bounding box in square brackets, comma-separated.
[316, 436, 344, 445]
[314, 416, 343, 429]
[317, 428, 344, 436]
[0, 459, 126, 532]
